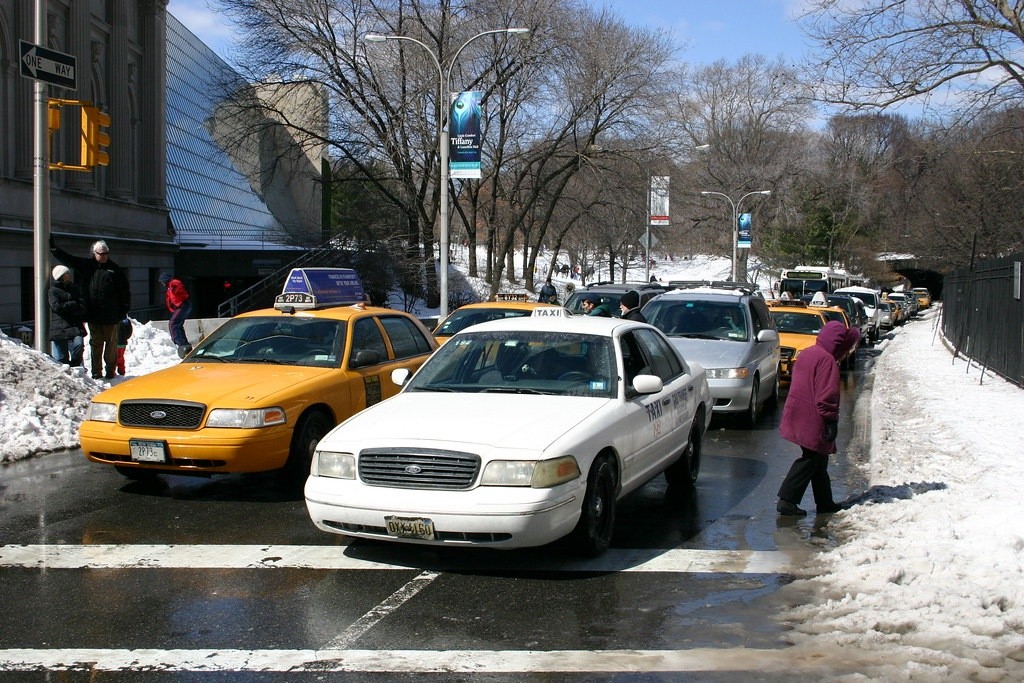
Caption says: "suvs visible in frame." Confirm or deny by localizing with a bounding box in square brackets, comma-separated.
[638, 280, 782, 431]
[560, 280, 672, 319]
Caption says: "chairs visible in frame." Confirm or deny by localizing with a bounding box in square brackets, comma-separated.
[676, 308, 712, 332]
[492, 338, 531, 381]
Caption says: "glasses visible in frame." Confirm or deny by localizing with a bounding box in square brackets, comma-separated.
[95, 251, 109, 256]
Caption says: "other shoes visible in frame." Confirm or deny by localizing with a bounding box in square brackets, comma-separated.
[817, 502, 842, 514]
[777, 499, 807, 516]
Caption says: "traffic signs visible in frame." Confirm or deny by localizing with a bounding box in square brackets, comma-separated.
[19, 39, 78, 91]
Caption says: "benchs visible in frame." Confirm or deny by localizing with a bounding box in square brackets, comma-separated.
[530, 354, 596, 378]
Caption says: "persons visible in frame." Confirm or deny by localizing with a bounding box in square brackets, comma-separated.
[620, 291, 647, 323]
[159, 272, 192, 346]
[580, 293, 613, 359]
[48, 264, 87, 366]
[648, 276, 662, 289]
[548, 295, 559, 306]
[538, 278, 556, 303]
[777, 321, 860, 516]
[48, 232, 132, 379]
[116, 318, 132, 376]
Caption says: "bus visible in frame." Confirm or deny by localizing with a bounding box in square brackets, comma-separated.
[774, 265, 850, 300]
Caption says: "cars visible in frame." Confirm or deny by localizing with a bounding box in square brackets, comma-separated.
[304, 306, 714, 558]
[431, 292, 575, 369]
[79, 267, 442, 490]
[762, 287, 933, 388]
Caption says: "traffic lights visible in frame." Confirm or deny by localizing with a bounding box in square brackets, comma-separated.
[80, 107, 110, 171]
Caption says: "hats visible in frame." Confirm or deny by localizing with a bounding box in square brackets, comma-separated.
[547, 278, 552, 283]
[159, 272, 172, 282]
[622, 291, 639, 309]
[580, 294, 601, 306]
[52, 265, 71, 280]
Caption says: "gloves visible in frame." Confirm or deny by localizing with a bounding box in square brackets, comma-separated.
[49, 233, 56, 249]
[824, 420, 837, 441]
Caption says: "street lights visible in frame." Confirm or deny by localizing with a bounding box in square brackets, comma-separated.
[584, 141, 711, 277]
[365, 26, 530, 327]
[703, 190, 771, 282]
